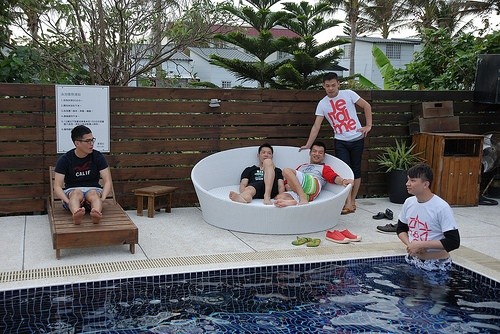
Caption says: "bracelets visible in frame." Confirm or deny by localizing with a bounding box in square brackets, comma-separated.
[342, 179, 347, 186]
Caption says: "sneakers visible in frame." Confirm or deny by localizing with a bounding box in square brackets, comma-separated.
[377, 224, 398, 234]
[326, 229, 362, 243]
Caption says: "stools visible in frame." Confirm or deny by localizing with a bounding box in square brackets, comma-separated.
[135, 185, 179, 218]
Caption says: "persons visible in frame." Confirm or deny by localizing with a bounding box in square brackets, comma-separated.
[299, 72, 373, 214]
[53, 125, 111, 225]
[229, 141, 355, 208]
[396, 164, 460, 272]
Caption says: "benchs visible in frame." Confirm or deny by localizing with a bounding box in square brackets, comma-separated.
[191, 145, 355, 235]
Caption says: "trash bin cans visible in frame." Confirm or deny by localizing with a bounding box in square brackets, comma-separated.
[411, 132, 484, 208]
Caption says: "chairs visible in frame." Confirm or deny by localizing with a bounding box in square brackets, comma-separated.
[482, 130, 500, 201]
[47, 166, 138, 260]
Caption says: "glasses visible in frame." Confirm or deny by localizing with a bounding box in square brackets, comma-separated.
[78, 137, 96, 143]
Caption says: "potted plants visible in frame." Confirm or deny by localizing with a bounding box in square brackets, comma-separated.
[375, 137, 428, 204]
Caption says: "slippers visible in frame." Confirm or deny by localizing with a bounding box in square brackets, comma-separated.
[292, 237, 321, 247]
[341, 205, 356, 215]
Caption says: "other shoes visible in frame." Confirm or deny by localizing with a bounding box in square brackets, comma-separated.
[372, 208, 393, 219]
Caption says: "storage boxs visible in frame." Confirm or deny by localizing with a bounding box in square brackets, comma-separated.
[409, 101, 460, 135]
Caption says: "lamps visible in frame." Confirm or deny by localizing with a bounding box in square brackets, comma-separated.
[208, 98, 221, 108]
